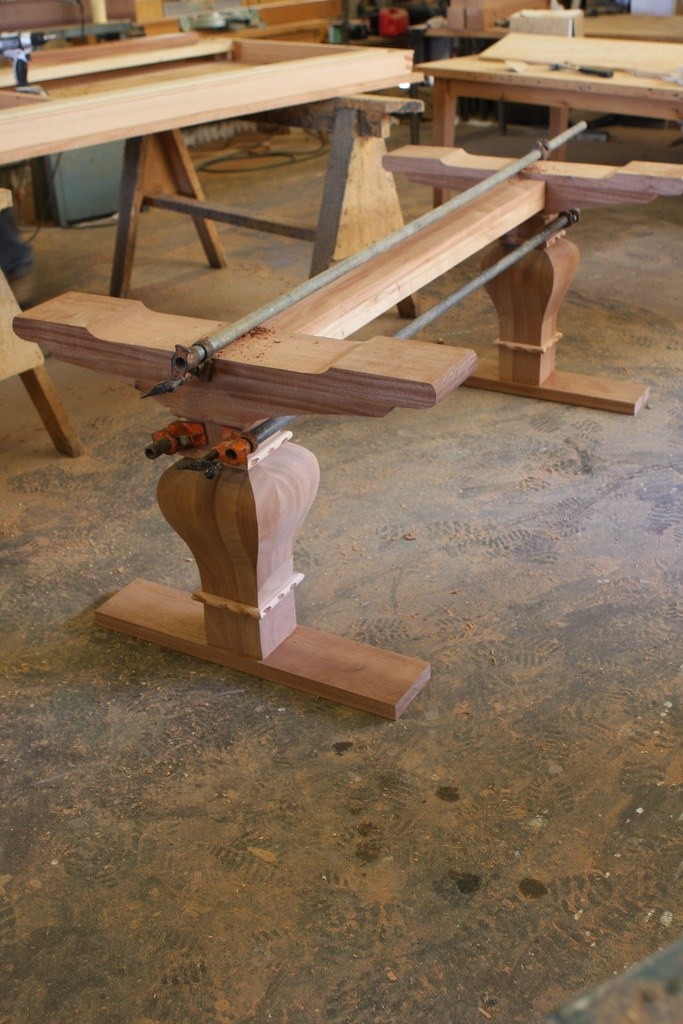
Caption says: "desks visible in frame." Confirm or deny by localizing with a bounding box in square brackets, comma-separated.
[0, 30, 425, 456]
[413, 13, 683, 211]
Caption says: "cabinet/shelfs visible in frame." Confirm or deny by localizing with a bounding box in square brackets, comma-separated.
[34, 139, 129, 228]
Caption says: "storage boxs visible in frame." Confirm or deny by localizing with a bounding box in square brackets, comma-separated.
[510, 8, 584, 38]
[447, 5, 483, 30]
